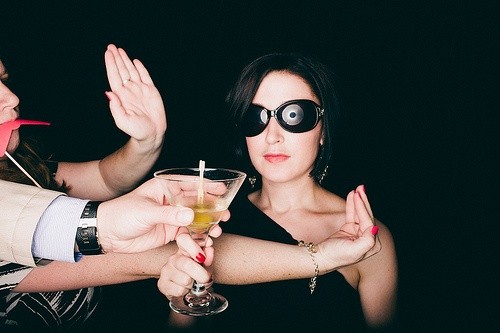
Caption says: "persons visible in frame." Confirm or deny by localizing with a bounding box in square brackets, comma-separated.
[0, 43, 383, 333]
[156, 53, 397, 333]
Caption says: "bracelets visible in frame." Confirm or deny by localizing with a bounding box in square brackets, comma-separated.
[75, 199, 105, 256]
[296, 240, 319, 294]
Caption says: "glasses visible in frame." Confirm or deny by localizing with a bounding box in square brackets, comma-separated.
[237, 102, 324, 137]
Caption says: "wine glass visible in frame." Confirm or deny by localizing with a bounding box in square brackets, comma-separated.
[154, 168, 247, 317]
